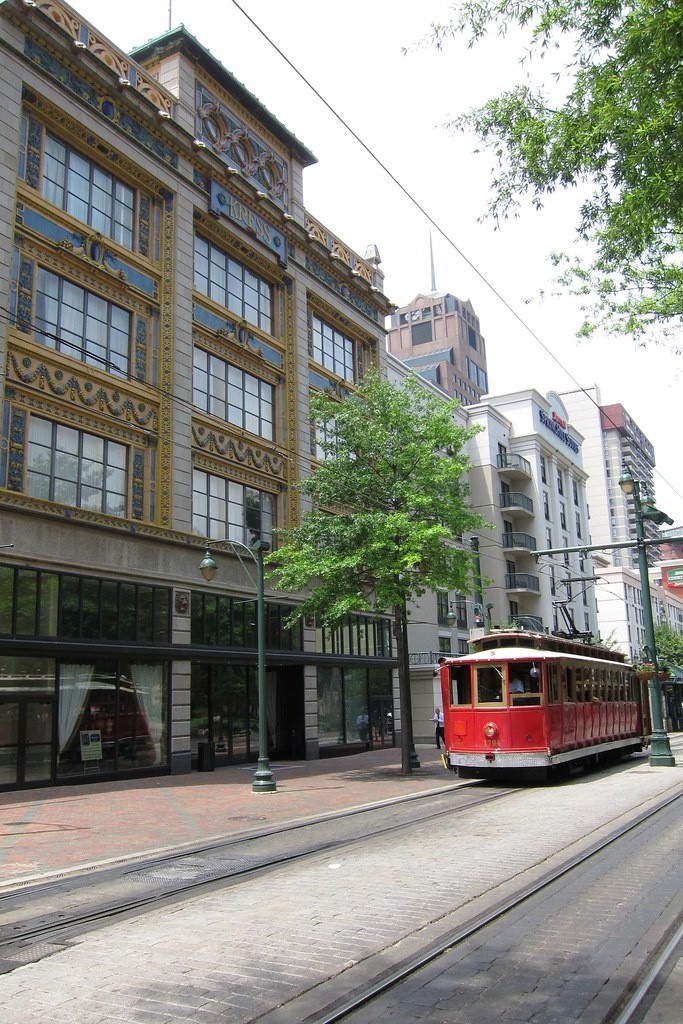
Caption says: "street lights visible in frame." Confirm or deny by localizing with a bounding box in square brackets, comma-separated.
[618, 456, 678, 766]
[197, 538, 277, 793]
[445, 599, 495, 634]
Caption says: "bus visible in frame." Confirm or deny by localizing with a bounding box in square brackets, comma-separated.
[1, 678, 161, 778]
[431, 574, 653, 784]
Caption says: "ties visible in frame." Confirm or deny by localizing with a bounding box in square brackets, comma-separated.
[362, 716, 364, 725]
[437, 714, 439, 727]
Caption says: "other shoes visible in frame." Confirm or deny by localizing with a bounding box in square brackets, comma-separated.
[435, 747, 441, 749]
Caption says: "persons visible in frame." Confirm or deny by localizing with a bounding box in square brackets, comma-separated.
[498, 671, 526, 702]
[357, 709, 370, 747]
[433, 708, 445, 749]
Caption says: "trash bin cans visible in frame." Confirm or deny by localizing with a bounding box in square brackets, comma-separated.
[197, 742, 215, 772]
